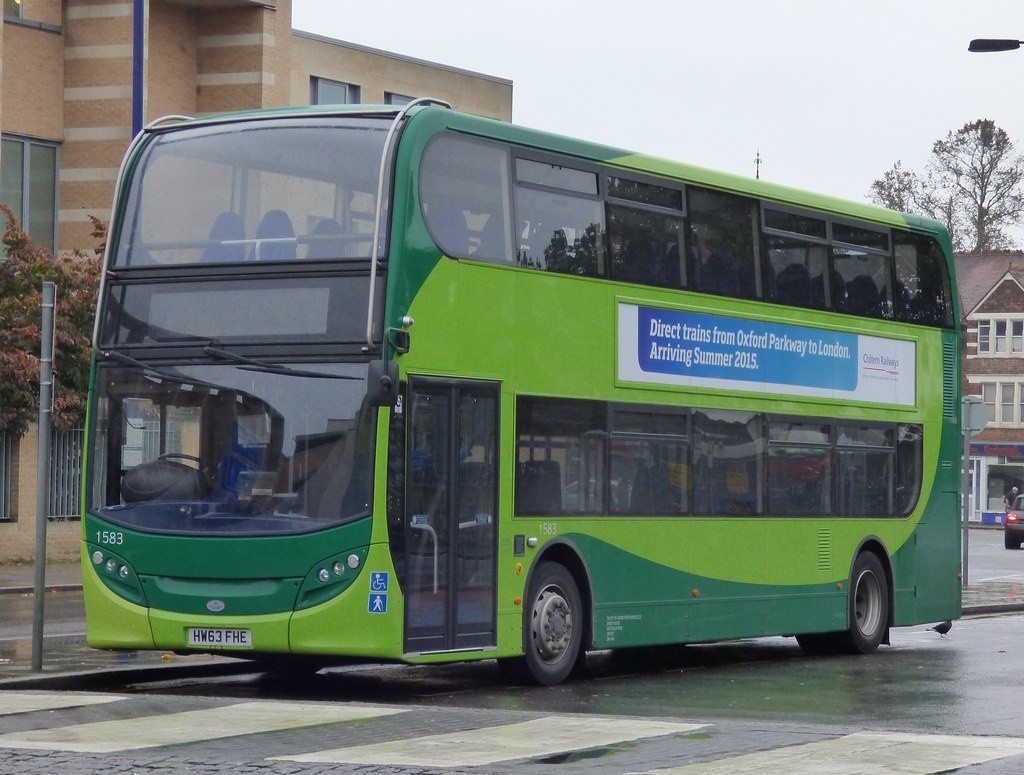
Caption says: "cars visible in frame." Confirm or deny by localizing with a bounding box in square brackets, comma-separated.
[1003, 494, 1024, 548]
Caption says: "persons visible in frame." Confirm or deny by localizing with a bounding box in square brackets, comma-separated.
[1004, 486, 1019, 514]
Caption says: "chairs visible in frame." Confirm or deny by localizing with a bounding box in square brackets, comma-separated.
[122, 204, 926, 541]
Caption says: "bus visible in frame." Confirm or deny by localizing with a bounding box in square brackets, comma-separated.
[77, 97, 969, 685]
